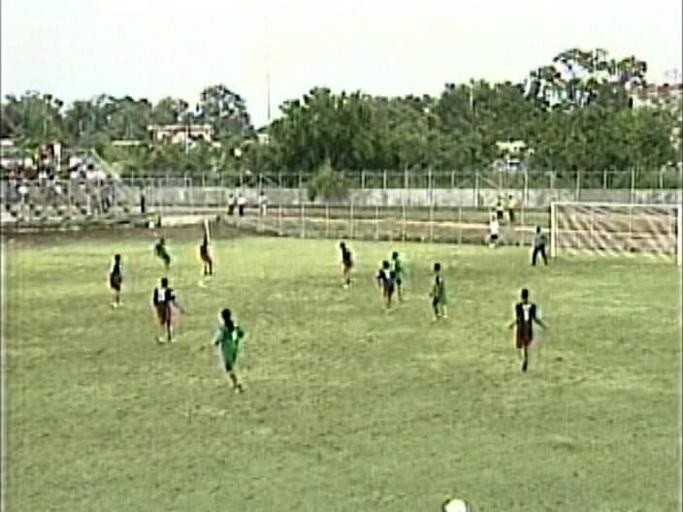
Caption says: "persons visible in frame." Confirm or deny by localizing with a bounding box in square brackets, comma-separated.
[154, 235, 170, 270]
[213, 308, 243, 392]
[199, 233, 214, 275]
[531, 224, 550, 267]
[226, 188, 269, 217]
[427, 263, 447, 322]
[152, 277, 185, 343]
[340, 242, 353, 289]
[489, 194, 517, 247]
[374, 251, 405, 309]
[508, 288, 549, 372]
[107, 253, 123, 307]
[3, 164, 113, 212]
